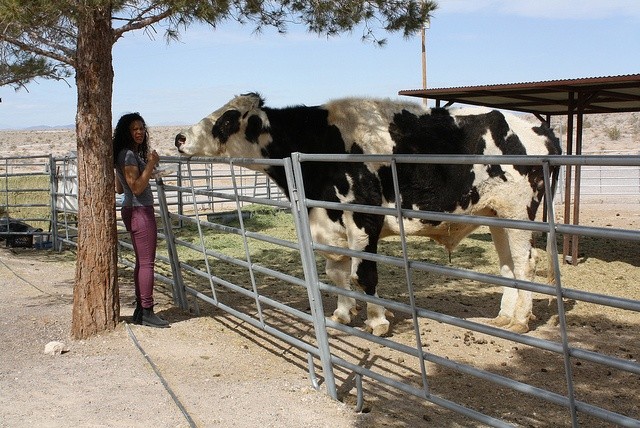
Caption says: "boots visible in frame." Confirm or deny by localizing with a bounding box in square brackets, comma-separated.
[135, 307, 169, 328]
[133, 308, 140, 320]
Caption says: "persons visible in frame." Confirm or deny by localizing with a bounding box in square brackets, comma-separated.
[113, 111, 170, 327]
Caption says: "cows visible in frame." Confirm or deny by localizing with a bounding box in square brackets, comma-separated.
[174, 89, 563, 336]
